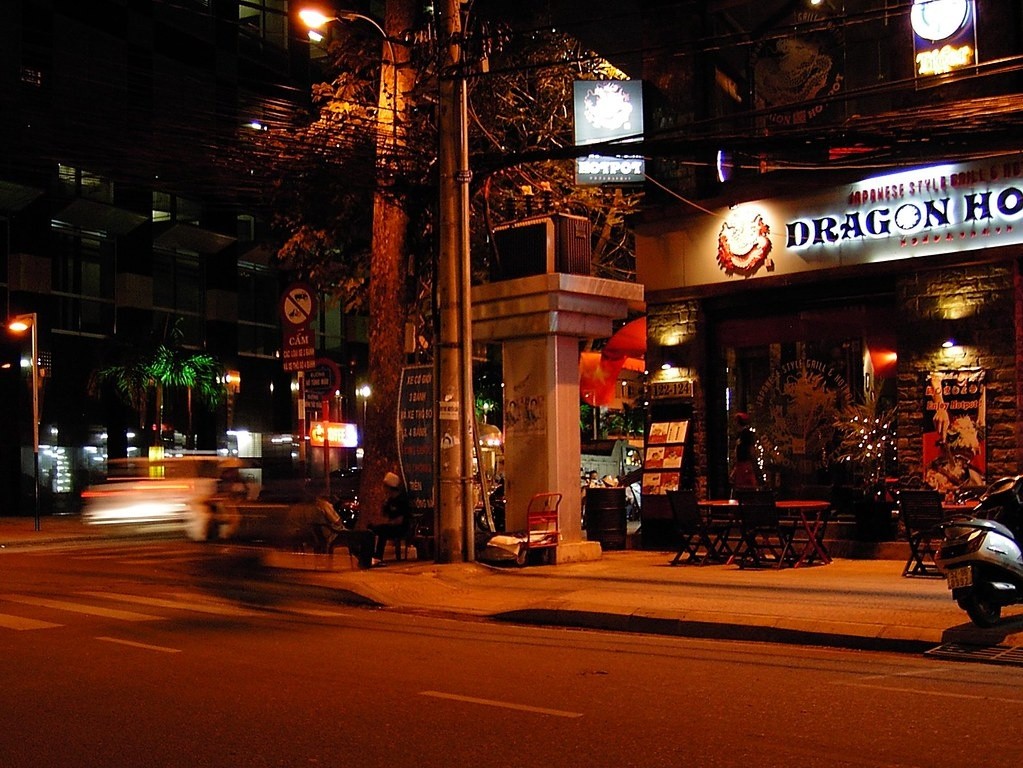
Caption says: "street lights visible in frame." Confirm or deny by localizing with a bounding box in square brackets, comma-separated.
[10, 313, 40, 532]
[298, 8, 397, 149]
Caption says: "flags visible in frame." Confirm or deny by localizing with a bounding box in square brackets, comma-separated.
[579, 352, 625, 406]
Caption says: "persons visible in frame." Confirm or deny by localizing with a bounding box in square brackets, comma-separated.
[580, 468, 641, 530]
[367, 472, 411, 561]
[476, 464, 487, 481]
[489, 456, 505, 535]
[729, 439, 775, 560]
[642, 425, 679, 494]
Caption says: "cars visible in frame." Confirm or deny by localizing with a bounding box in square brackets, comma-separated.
[329, 466, 362, 527]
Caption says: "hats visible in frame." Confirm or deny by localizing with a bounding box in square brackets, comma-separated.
[382, 473, 399, 487]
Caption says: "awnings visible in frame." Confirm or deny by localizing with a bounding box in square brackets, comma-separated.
[618, 357, 645, 379]
[600, 316, 647, 362]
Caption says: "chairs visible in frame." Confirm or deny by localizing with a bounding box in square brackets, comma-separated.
[306, 522, 362, 560]
[400, 512, 425, 559]
[900, 489, 974, 580]
[664, 490, 831, 568]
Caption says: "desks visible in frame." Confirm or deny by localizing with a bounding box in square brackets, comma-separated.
[770, 499, 832, 568]
[696, 499, 765, 564]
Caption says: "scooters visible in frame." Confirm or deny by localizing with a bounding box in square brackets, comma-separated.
[934, 475, 1023, 628]
[476, 477, 506, 533]
